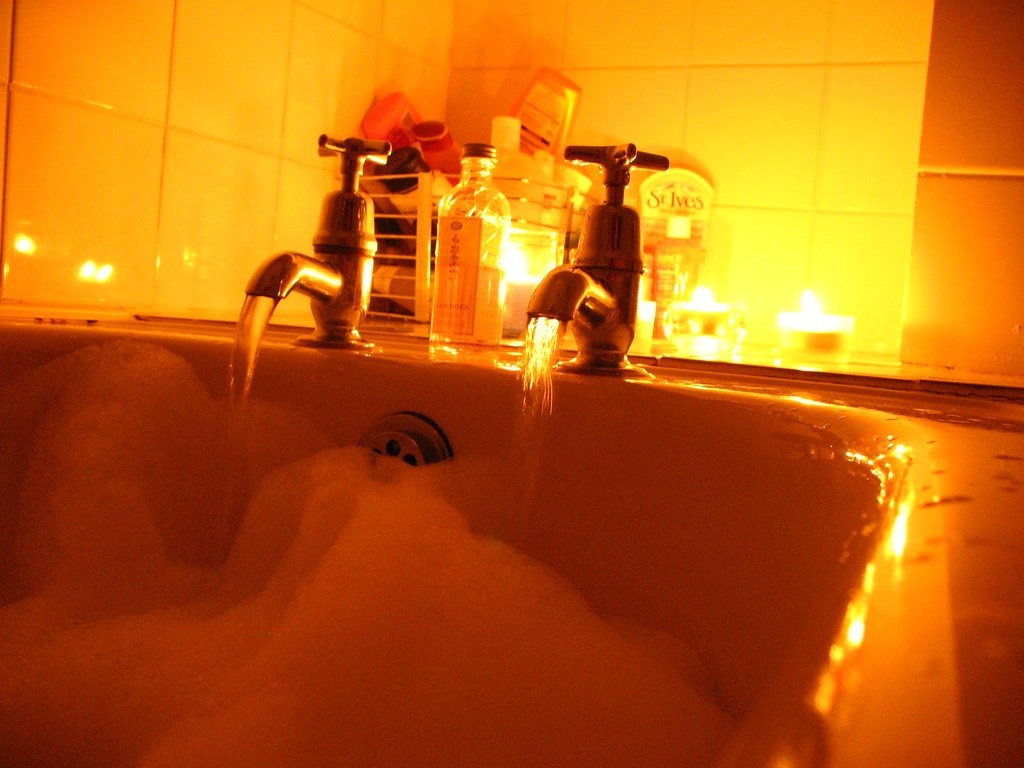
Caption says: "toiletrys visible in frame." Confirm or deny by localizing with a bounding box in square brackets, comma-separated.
[427, 143, 511, 363]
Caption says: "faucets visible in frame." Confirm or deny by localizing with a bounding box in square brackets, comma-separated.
[243, 133, 394, 350]
[525, 143, 670, 378]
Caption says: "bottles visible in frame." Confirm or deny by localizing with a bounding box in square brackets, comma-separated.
[429, 149, 506, 366]
[486, 113, 533, 218]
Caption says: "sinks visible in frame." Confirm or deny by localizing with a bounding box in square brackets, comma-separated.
[0, 323, 917, 768]
